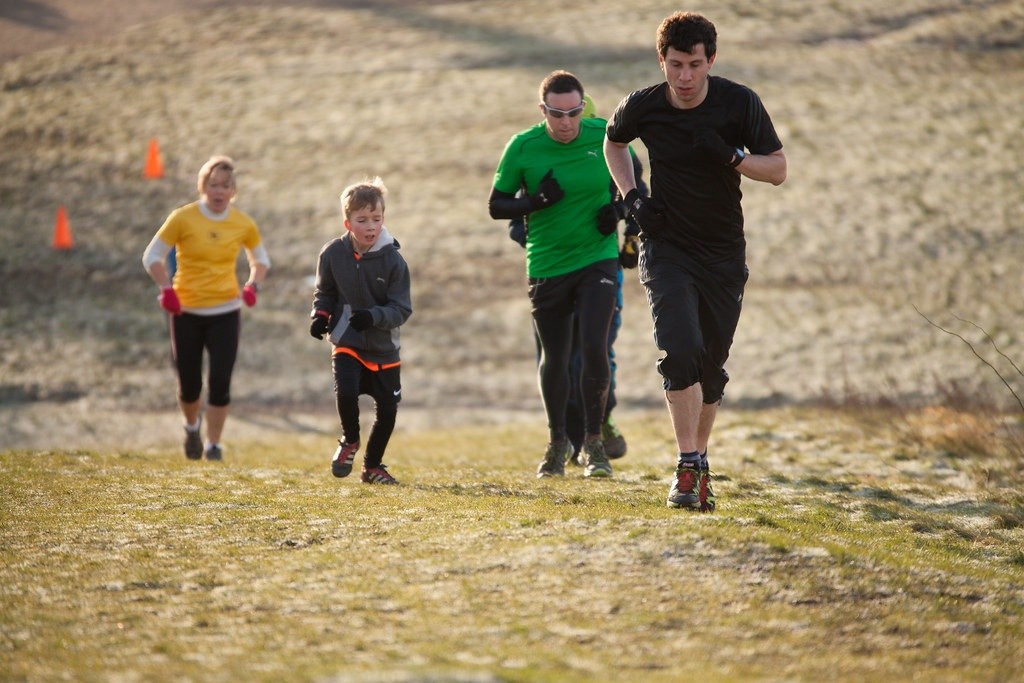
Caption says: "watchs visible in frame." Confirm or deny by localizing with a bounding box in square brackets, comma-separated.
[729, 147, 745, 168]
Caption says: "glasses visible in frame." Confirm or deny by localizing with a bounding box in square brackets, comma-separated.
[544, 104, 583, 118]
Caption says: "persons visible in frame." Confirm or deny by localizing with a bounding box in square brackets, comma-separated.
[603, 13, 786, 511]
[310, 183, 412, 485]
[489, 70, 649, 478]
[142, 155, 269, 460]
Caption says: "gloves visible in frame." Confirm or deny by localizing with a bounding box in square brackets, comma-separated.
[598, 203, 624, 236]
[160, 287, 181, 315]
[620, 240, 639, 269]
[529, 169, 563, 210]
[630, 195, 666, 237]
[691, 126, 735, 165]
[242, 284, 256, 306]
[349, 310, 373, 332]
[309, 315, 329, 340]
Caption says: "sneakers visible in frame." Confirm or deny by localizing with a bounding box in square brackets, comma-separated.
[577, 439, 612, 477]
[206, 445, 221, 460]
[667, 468, 701, 509]
[537, 435, 575, 479]
[184, 414, 203, 460]
[331, 435, 360, 478]
[601, 416, 626, 458]
[360, 463, 400, 486]
[699, 470, 717, 514]
